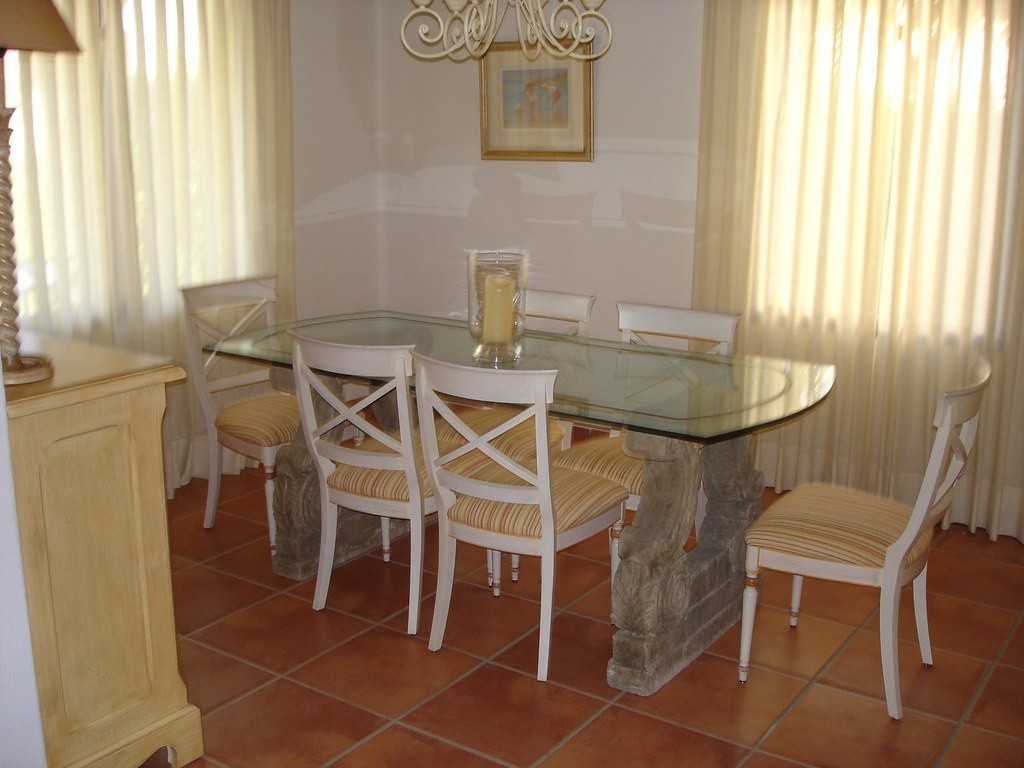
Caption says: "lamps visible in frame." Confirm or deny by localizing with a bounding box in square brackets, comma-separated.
[0, 0, 81, 386]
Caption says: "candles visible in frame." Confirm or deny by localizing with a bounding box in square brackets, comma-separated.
[481, 273, 516, 346]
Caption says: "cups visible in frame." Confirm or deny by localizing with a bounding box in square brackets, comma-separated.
[467, 252, 528, 364]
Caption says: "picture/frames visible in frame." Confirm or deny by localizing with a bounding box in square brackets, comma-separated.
[480, 39, 594, 161]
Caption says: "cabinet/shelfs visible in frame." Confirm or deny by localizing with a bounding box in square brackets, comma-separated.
[0, 329, 204, 768]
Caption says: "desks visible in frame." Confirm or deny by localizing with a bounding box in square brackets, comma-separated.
[200, 310, 835, 699]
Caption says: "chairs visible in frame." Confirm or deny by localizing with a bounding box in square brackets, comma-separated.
[552, 302, 741, 547]
[415, 291, 597, 584]
[739, 352, 993, 716]
[407, 350, 628, 682]
[179, 273, 365, 565]
[287, 329, 509, 636]
